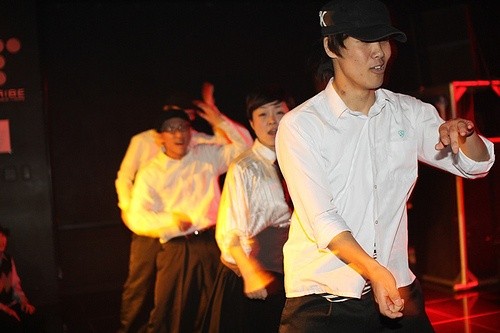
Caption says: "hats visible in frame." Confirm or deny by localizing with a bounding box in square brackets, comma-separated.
[156, 108, 190, 134]
[317, 0, 407, 42]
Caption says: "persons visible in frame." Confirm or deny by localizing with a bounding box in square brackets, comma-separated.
[274, 0, 495, 333]
[0, 226, 40, 333]
[115, 81, 253, 333]
[205, 85, 294, 333]
